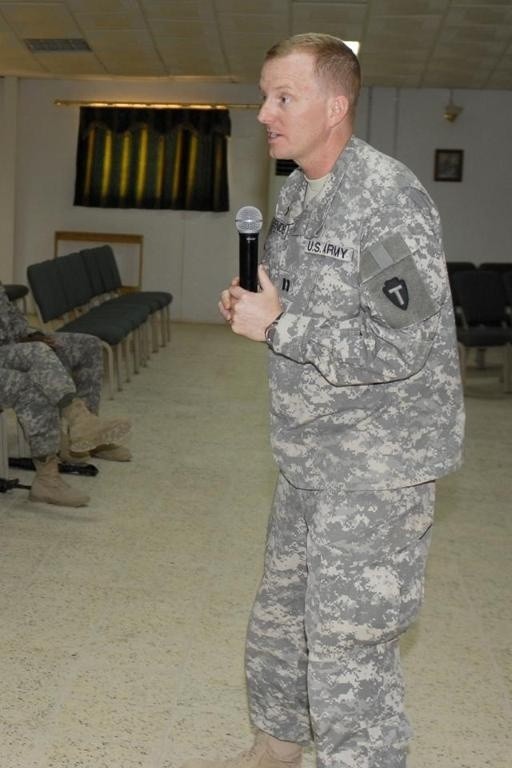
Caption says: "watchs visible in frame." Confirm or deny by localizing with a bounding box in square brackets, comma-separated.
[264, 307, 286, 348]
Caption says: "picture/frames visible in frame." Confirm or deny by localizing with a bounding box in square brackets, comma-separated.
[434, 149, 463, 182]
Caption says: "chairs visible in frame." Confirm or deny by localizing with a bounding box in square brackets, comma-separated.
[25, 244, 174, 401]
[446, 261, 512, 393]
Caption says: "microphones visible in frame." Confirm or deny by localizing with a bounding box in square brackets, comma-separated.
[234, 204, 261, 292]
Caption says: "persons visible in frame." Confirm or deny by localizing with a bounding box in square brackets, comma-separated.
[216, 29, 468, 768]
[0, 340, 135, 509]
[0, 278, 135, 464]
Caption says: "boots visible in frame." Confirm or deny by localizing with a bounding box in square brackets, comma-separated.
[60, 419, 91, 467]
[62, 399, 135, 453]
[91, 445, 132, 461]
[184, 730, 303, 768]
[28, 456, 89, 507]
